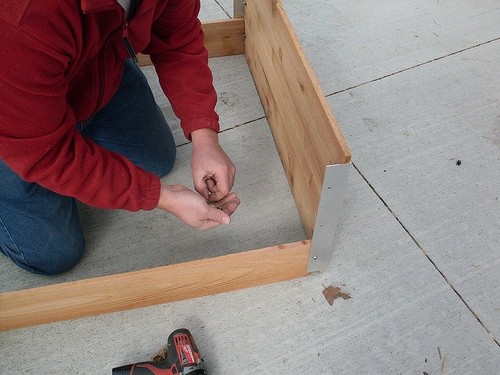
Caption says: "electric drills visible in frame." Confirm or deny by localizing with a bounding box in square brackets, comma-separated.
[112, 326, 208, 375]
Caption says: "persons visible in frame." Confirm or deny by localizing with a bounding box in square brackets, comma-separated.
[0, 0, 239, 276]
